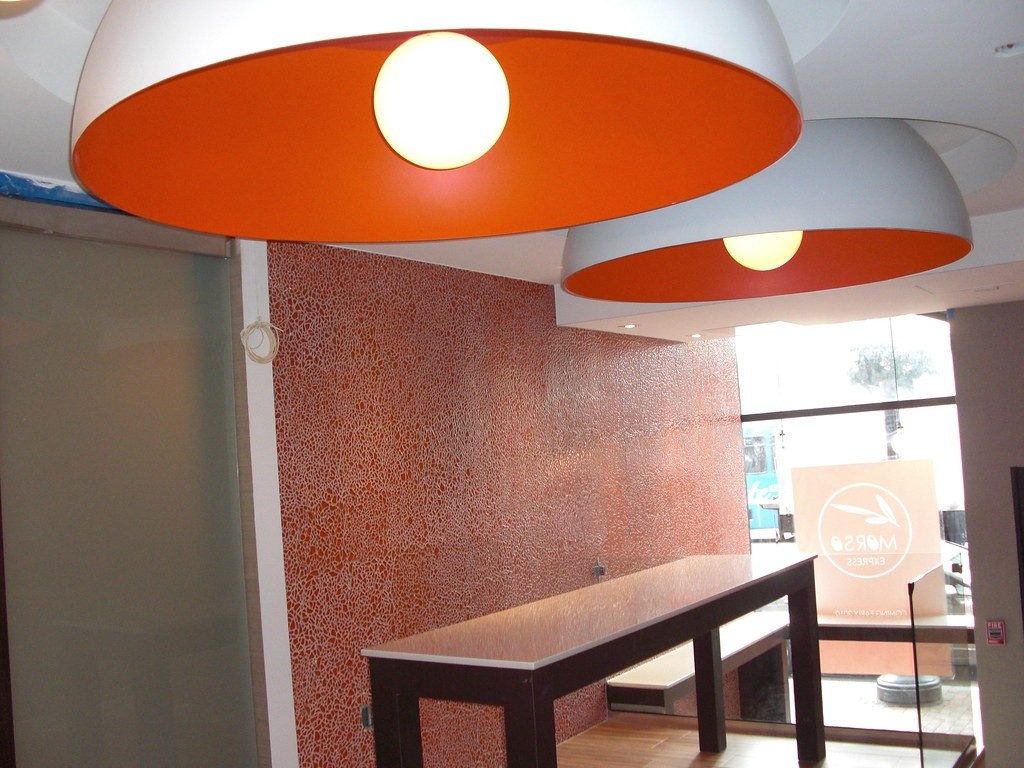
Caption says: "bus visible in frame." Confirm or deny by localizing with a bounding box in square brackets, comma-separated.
[743, 428, 780, 532]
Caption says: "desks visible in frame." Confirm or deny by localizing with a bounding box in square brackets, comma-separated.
[360, 554, 825, 768]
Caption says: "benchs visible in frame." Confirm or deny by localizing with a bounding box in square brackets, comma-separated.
[605, 608, 789, 724]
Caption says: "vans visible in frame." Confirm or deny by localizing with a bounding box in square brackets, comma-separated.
[940, 469, 974, 585]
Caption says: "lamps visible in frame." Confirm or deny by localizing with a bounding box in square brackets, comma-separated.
[561, 118, 974, 303]
[70, 0, 802, 243]
